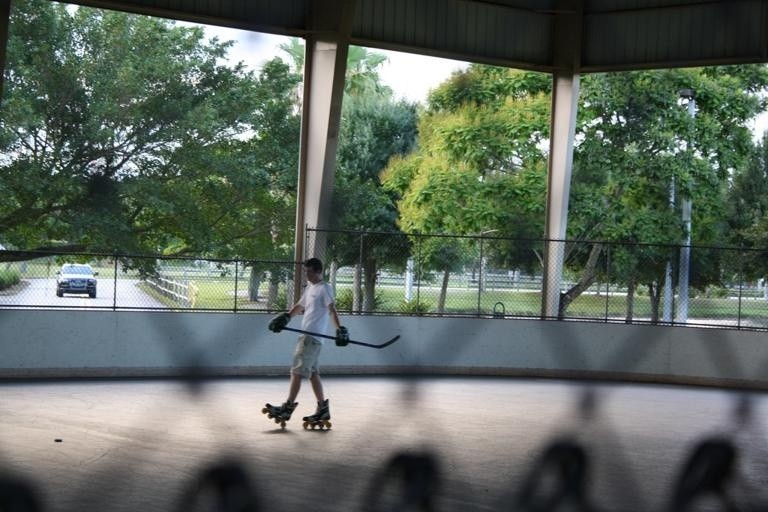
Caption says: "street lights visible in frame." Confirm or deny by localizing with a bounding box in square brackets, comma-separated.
[674, 88, 697, 323]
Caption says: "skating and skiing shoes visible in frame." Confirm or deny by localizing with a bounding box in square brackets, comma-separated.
[261, 399, 299, 428]
[302, 400, 333, 429]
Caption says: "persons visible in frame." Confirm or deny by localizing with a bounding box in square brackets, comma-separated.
[264, 258, 350, 423]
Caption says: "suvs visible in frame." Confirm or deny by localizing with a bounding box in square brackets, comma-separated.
[52, 262, 99, 300]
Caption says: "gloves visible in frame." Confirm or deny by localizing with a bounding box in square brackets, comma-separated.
[335, 324, 350, 347]
[268, 313, 291, 334]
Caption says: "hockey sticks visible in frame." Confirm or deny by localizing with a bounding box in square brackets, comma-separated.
[282, 327, 401, 349]
